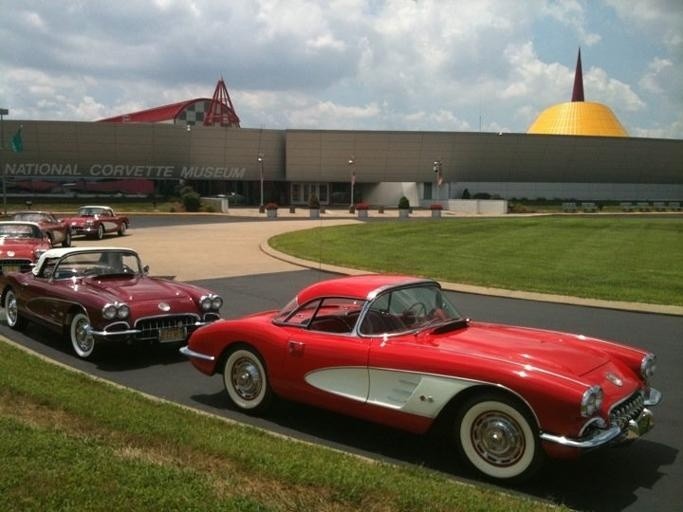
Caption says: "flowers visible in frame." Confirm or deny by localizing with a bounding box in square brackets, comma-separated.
[356, 202, 368, 209]
[431, 202, 443, 210]
[266, 203, 278, 209]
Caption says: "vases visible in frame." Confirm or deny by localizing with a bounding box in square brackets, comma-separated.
[359, 210, 368, 218]
[432, 212, 439, 218]
[267, 211, 276, 218]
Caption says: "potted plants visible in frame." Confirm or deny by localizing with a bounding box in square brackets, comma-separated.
[398, 196, 409, 218]
[309, 192, 320, 218]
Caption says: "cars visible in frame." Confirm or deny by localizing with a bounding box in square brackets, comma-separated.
[12, 209, 74, 248]
[0, 219, 52, 275]
[179, 272, 664, 493]
[0, 245, 224, 362]
[65, 205, 130, 241]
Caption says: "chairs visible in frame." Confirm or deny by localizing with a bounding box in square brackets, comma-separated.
[307, 317, 352, 334]
[348, 308, 394, 334]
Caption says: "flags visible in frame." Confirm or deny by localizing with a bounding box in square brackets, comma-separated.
[437, 168, 444, 187]
[8, 125, 24, 154]
[350, 168, 356, 186]
